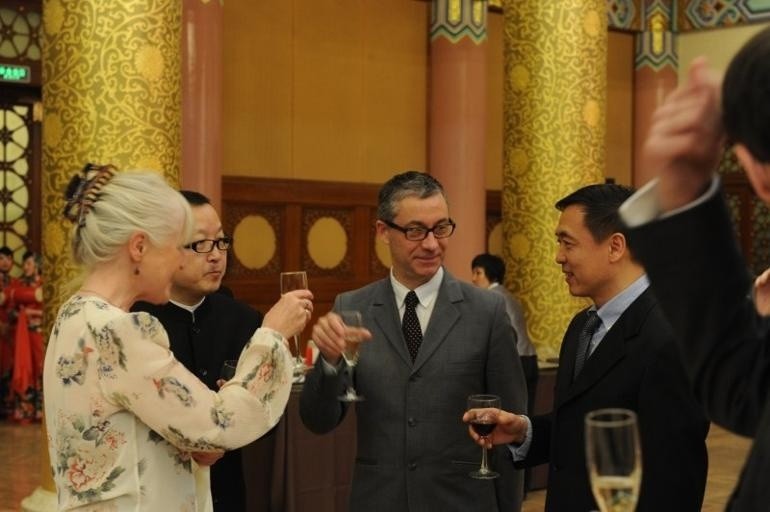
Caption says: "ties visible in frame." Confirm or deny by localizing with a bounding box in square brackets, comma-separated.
[570, 310, 601, 382]
[401, 290, 423, 363]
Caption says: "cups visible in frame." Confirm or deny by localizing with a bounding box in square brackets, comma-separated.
[585, 406, 643, 512]
[220, 360, 237, 390]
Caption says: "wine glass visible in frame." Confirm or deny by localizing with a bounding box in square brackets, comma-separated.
[466, 394, 501, 481]
[278, 271, 314, 374]
[337, 311, 365, 403]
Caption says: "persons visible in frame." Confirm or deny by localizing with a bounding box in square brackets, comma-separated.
[462, 182, 710, 512]
[0, 246, 43, 424]
[616, 22, 770, 512]
[41, 162, 314, 512]
[470, 252, 540, 501]
[129, 190, 264, 512]
[299, 170, 530, 512]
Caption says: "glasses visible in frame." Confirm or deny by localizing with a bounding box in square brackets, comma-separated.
[184, 234, 233, 253]
[386, 217, 456, 242]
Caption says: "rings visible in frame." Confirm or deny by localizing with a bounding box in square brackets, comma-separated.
[303, 300, 309, 309]
